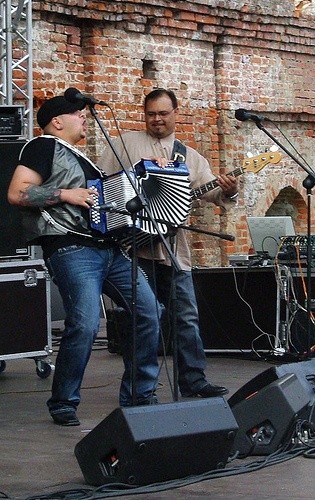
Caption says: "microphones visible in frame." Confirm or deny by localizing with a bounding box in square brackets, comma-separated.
[235, 108, 271, 121]
[93, 202, 117, 212]
[64, 88, 107, 106]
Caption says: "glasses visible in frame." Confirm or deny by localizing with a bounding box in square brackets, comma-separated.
[144, 108, 177, 120]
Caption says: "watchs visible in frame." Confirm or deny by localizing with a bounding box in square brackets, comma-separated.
[225, 190, 239, 200]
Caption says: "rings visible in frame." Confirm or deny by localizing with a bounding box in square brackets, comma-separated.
[86, 197, 91, 203]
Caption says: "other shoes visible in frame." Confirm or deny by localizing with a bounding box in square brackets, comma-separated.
[136, 398, 159, 405]
[191, 383, 230, 399]
[51, 410, 81, 426]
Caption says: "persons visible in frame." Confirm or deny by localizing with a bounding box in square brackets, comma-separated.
[94, 89, 239, 398]
[7, 96, 170, 427]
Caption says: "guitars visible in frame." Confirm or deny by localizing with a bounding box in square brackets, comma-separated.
[133, 150, 284, 262]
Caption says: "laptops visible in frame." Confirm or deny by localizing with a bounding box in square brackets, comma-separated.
[246, 216, 295, 258]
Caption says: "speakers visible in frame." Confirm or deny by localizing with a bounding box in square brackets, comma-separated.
[50, 278, 106, 330]
[74, 357, 315, 492]
[0, 138, 34, 259]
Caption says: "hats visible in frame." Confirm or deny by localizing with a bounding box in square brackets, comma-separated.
[36, 95, 87, 130]
